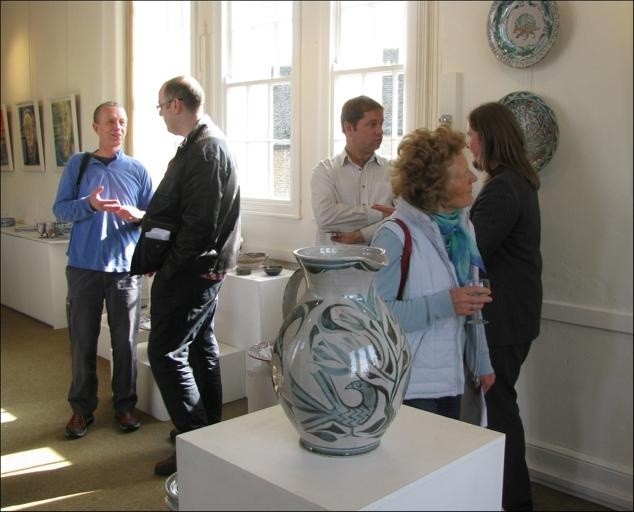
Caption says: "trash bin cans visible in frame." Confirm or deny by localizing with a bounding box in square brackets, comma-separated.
[246, 338, 279, 412]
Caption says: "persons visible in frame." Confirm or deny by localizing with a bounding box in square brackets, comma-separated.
[367, 121, 498, 420]
[309, 94, 402, 246]
[52, 101, 76, 167]
[130, 75, 243, 477]
[370, 103, 544, 511]
[22, 108, 39, 165]
[52, 101, 156, 438]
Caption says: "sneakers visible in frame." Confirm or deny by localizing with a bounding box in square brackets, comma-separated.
[114, 411, 141, 431]
[155, 454, 176, 475]
[66, 415, 93, 438]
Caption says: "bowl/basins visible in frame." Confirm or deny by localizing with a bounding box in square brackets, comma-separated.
[263, 266, 282, 276]
[236, 252, 269, 270]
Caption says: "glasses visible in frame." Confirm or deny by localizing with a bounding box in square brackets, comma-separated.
[157, 98, 182, 109]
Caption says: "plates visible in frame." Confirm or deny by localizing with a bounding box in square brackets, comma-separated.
[486, 1, 561, 68]
[499, 92, 559, 175]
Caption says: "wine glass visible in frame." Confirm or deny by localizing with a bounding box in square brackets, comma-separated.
[466, 279, 490, 325]
[36, 222, 55, 240]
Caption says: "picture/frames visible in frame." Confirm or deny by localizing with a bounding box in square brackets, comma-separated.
[14, 99, 45, 172]
[0, 105, 13, 172]
[47, 94, 80, 172]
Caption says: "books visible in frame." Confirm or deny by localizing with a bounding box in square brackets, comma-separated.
[15, 224, 39, 231]
